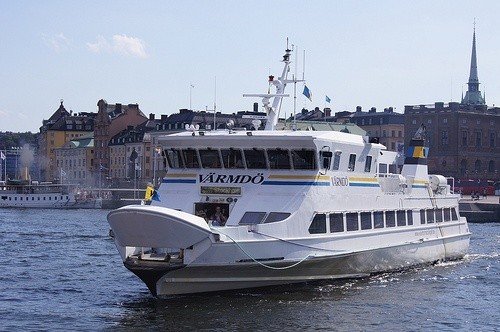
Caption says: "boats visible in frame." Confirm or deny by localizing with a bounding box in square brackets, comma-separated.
[106, 37, 474, 304]
[0, 175, 103, 210]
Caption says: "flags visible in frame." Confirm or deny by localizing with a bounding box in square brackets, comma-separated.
[326, 96, 331, 103]
[303, 86, 312, 101]
[146, 187, 161, 202]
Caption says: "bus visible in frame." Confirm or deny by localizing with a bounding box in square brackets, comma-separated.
[447, 177, 495, 196]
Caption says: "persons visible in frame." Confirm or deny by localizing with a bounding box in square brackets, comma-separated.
[483, 188, 488, 199]
[458, 187, 464, 198]
[211, 207, 226, 225]
[471, 191, 480, 198]
